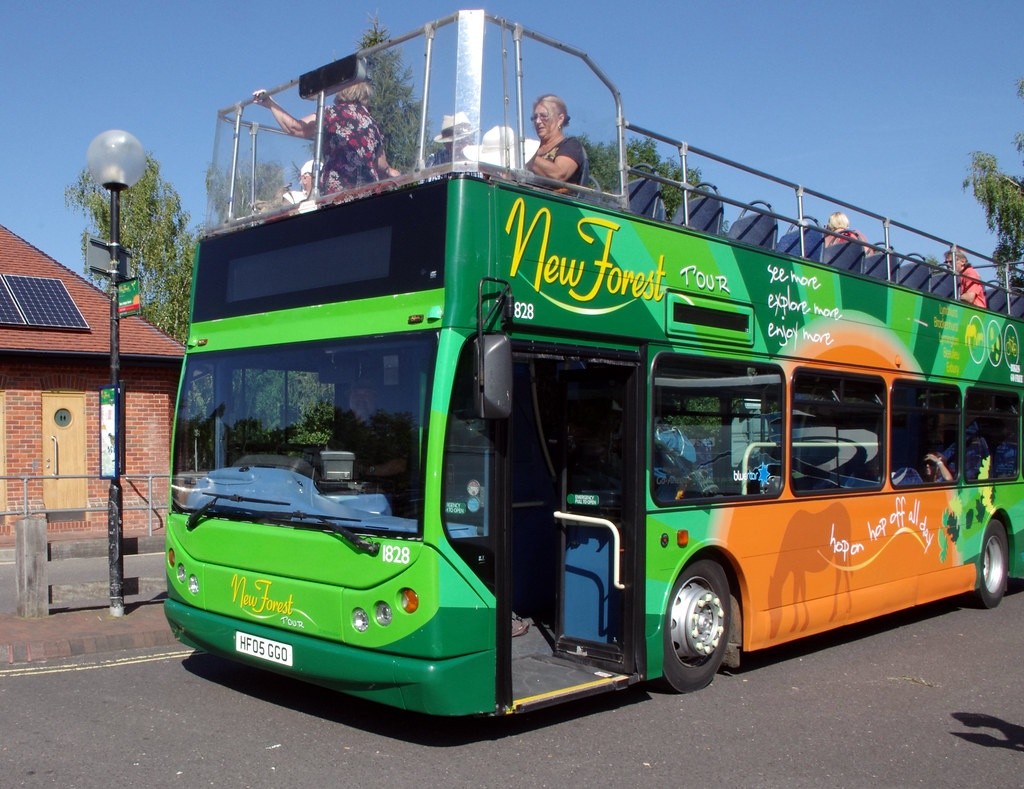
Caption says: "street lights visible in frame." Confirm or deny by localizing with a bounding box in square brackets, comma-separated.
[86, 128, 145, 616]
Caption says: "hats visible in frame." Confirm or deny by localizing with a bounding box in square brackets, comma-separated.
[301, 160, 323, 176]
[433, 111, 479, 143]
[462, 126, 540, 170]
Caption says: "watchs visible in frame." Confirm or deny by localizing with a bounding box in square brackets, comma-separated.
[369, 465, 376, 475]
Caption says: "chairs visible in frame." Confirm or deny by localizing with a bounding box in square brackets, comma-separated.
[994, 441, 1018, 477]
[893, 467, 921, 486]
[556, 137, 1024, 319]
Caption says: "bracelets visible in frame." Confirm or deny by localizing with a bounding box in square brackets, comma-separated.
[936, 461, 942, 466]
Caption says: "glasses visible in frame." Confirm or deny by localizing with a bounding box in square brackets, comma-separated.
[946, 261, 952, 263]
[531, 115, 550, 121]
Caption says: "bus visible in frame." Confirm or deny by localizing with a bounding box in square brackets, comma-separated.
[162, 8, 1024, 718]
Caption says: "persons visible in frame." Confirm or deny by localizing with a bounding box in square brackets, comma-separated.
[325, 375, 411, 515]
[253, 82, 400, 222]
[825, 211, 874, 256]
[944, 249, 986, 308]
[413, 94, 584, 197]
[924, 452, 953, 483]
